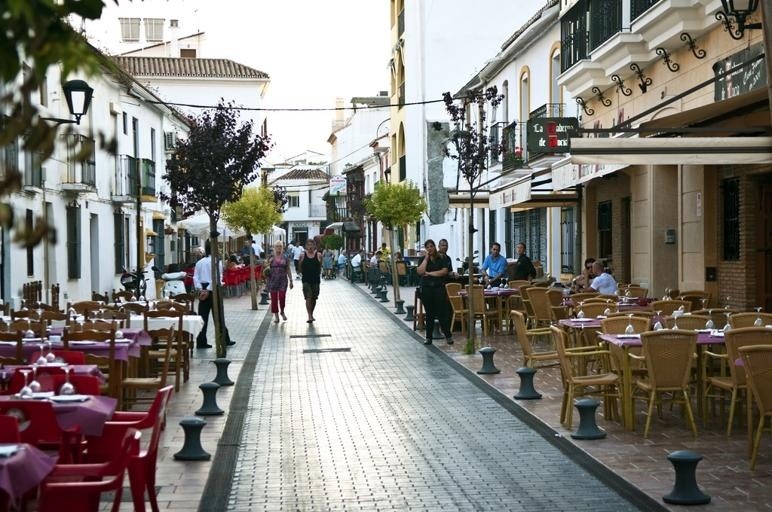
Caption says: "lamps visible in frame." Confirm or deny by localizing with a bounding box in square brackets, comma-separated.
[721, 0, 764, 33]
[40, 79, 95, 127]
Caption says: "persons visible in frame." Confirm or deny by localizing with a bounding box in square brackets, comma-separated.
[570, 257, 598, 290]
[437, 241, 459, 337]
[191, 239, 236, 350]
[298, 240, 323, 324]
[263, 240, 294, 323]
[481, 243, 509, 288]
[511, 242, 536, 281]
[411, 239, 454, 346]
[165, 240, 479, 293]
[582, 260, 617, 294]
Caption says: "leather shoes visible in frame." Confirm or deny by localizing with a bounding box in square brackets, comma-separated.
[227, 340, 236, 346]
[197, 344, 212, 348]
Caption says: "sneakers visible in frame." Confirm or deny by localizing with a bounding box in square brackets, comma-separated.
[446, 337, 454, 345]
[423, 337, 432, 344]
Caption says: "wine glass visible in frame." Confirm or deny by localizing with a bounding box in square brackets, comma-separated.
[1, 288, 176, 400]
[486, 277, 771, 337]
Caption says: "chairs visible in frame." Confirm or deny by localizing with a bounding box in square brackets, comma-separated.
[155, 256, 259, 299]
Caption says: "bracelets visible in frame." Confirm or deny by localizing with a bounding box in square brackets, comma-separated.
[489, 279, 491, 282]
